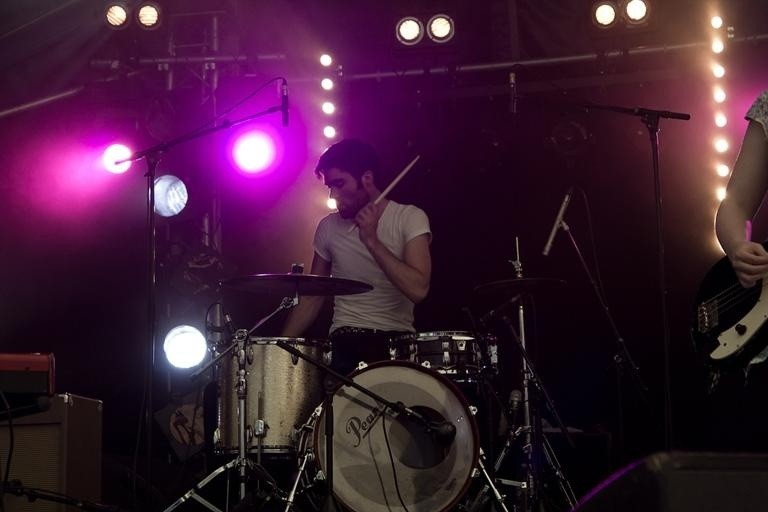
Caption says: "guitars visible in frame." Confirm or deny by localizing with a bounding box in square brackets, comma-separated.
[690, 241, 767, 369]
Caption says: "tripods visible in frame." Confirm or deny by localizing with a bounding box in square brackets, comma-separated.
[471, 283, 580, 512]
[164, 298, 298, 512]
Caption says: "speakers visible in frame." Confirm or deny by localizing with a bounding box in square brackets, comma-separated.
[569, 454, 768, 512]
[0, 393, 104, 512]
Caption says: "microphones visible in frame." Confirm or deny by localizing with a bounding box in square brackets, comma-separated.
[541, 190, 573, 255]
[480, 293, 522, 322]
[402, 405, 456, 441]
[508, 71, 519, 115]
[282, 84, 289, 127]
[220, 305, 235, 335]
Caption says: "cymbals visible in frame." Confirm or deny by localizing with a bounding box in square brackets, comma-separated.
[467, 276, 566, 307]
[218, 273, 373, 296]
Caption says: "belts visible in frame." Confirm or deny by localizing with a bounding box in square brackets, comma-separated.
[329, 326, 390, 337]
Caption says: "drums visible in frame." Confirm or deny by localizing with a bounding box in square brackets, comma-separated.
[292, 359, 480, 511]
[213, 335, 333, 461]
[387, 330, 500, 384]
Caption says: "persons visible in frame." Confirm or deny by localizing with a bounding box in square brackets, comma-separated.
[711, 89, 767, 454]
[282, 136, 432, 381]
